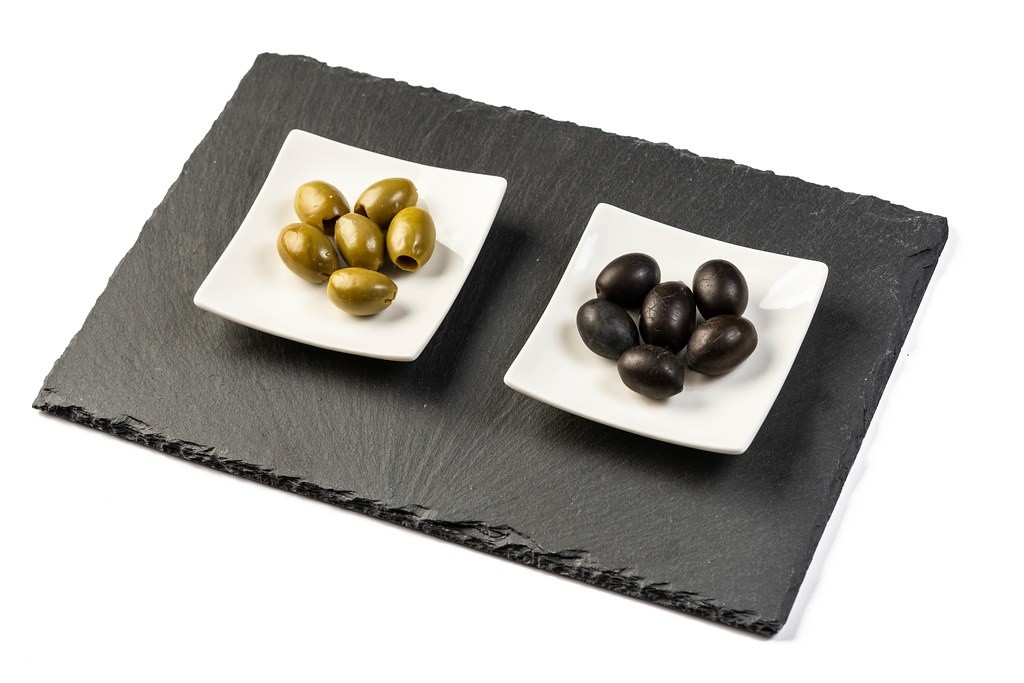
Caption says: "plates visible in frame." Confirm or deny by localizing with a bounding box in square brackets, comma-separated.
[503, 203, 829, 456]
[193, 128, 508, 362]
